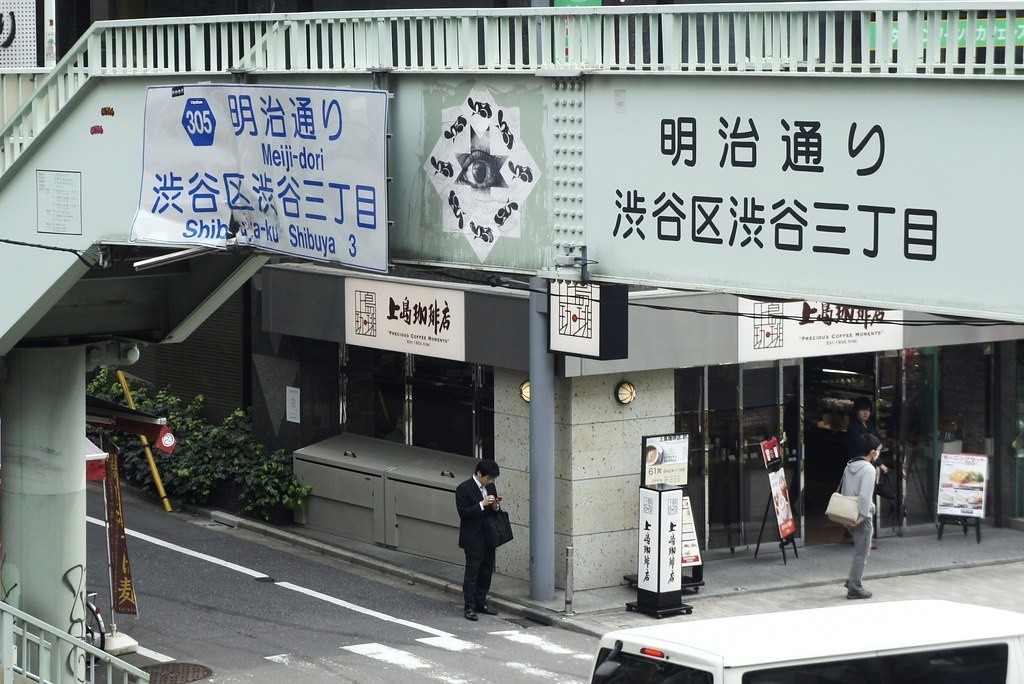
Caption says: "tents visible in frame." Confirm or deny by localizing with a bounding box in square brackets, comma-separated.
[85, 437, 117, 637]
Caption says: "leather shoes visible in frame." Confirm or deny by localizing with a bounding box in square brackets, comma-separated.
[477, 605, 498, 615]
[465, 609, 478, 621]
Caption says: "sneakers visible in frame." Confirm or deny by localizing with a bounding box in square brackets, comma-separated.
[845, 580, 864, 590]
[847, 590, 872, 599]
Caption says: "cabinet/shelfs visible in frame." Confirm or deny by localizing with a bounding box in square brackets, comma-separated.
[810, 358, 893, 432]
[293, 432, 484, 566]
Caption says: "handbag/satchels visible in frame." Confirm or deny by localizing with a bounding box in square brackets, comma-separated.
[875, 472, 897, 499]
[825, 492, 860, 525]
[484, 501, 514, 550]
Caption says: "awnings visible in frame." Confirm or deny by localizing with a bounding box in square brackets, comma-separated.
[84, 394, 176, 454]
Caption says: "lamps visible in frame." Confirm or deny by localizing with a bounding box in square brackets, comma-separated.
[614, 381, 636, 404]
[519, 380, 530, 402]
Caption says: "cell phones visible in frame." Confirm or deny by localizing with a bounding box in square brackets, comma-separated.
[496, 496, 502, 501]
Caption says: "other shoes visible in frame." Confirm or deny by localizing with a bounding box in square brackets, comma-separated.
[843, 536, 854, 544]
[870, 543, 877, 549]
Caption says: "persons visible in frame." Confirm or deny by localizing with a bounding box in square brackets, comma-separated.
[455, 459, 504, 621]
[841, 434, 883, 600]
[843, 397, 888, 549]
